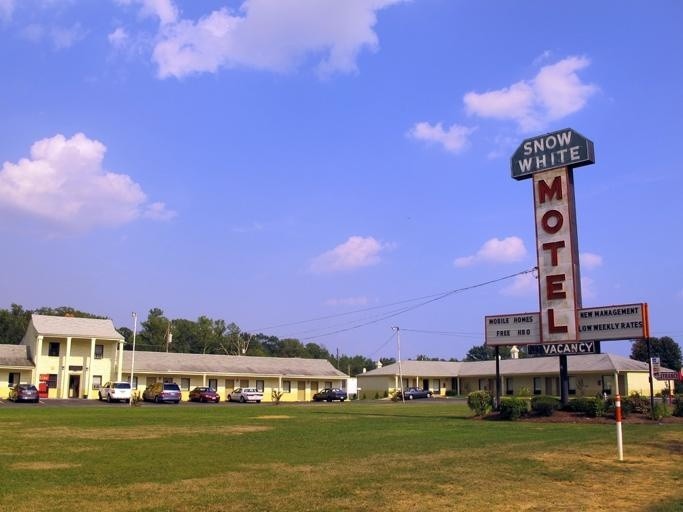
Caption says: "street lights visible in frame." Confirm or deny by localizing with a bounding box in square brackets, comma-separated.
[392, 326, 400, 362]
[130, 312, 137, 403]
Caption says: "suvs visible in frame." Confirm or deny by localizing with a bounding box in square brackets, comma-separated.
[143, 382, 182, 404]
[98, 381, 130, 403]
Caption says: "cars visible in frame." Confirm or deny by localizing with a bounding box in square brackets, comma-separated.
[189, 387, 220, 403]
[397, 387, 432, 400]
[227, 388, 263, 403]
[9, 384, 39, 403]
[313, 388, 347, 402]
